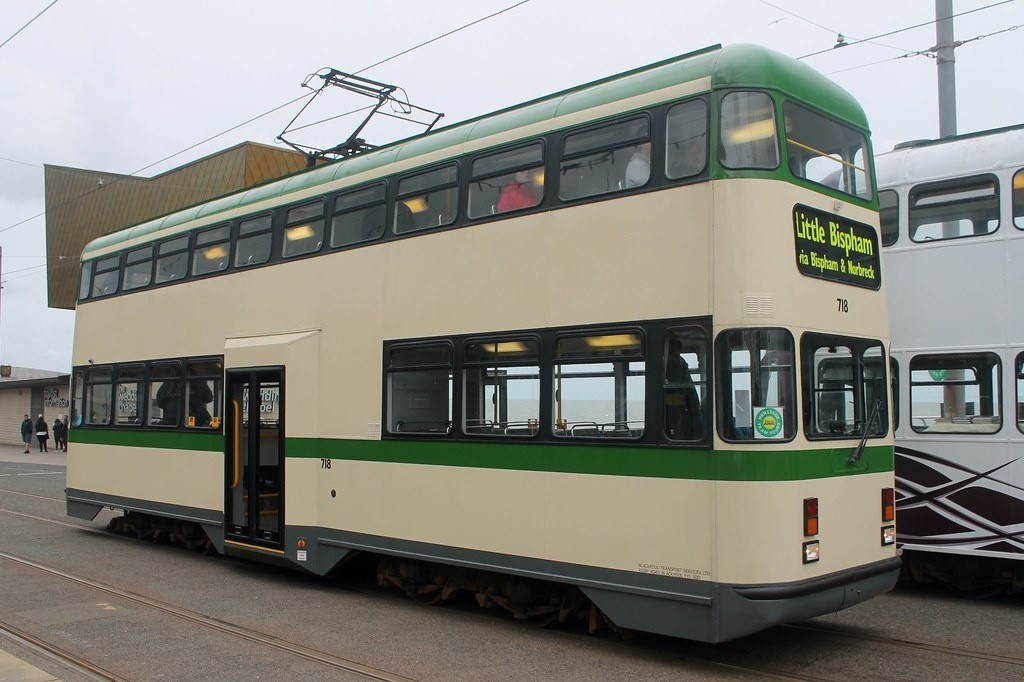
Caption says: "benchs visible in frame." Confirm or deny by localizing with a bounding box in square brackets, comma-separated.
[134, 417, 163, 424]
[396, 418, 645, 438]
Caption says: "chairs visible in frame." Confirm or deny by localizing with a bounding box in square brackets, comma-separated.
[102, 179, 624, 295]
[924, 236, 935, 241]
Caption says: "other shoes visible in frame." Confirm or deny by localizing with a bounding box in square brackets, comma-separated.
[45, 451, 48, 452]
[40, 450, 42, 452]
[24, 450, 29, 454]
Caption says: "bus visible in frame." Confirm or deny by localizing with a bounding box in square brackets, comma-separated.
[61, 39, 907, 644]
[440, 118, 1024, 573]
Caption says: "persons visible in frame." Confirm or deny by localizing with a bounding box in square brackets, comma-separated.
[20, 415, 33, 454]
[35, 416, 49, 454]
[669, 141, 701, 179]
[667, 337, 705, 440]
[624, 125, 651, 189]
[61, 415, 68, 452]
[53, 419, 63, 452]
[157, 367, 213, 427]
[497, 162, 538, 212]
